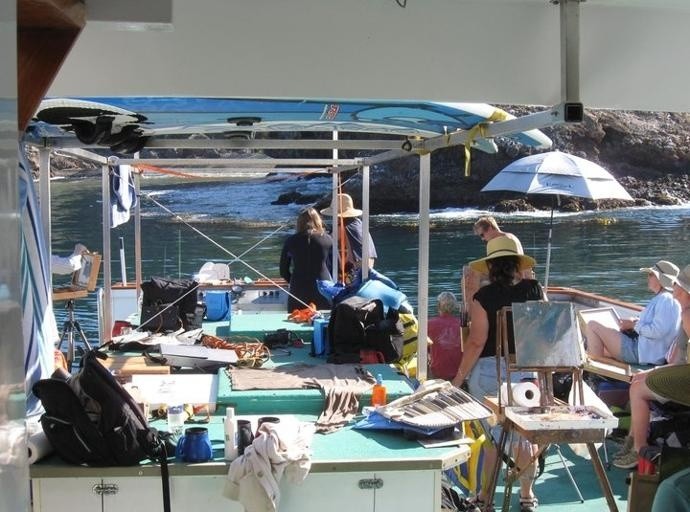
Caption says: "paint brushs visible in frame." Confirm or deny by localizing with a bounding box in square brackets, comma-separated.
[64, 235, 89, 264]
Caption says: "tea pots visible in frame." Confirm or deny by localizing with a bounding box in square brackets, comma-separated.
[175, 426, 212, 461]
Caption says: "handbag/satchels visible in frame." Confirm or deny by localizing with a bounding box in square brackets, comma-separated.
[139, 299, 180, 333]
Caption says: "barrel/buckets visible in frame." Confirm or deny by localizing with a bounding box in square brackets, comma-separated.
[204, 289, 232, 321]
[5, 387, 26, 421]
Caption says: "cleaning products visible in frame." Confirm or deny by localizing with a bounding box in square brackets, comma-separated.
[371, 374, 387, 405]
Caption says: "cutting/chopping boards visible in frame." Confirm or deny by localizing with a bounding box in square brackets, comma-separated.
[96, 354, 171, 374]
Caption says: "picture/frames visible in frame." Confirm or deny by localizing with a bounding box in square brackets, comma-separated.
[571, 299, 636, 385]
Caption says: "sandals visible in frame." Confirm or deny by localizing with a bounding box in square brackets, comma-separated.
[467, 495, 497, 506]
[518, 492, 539, 510]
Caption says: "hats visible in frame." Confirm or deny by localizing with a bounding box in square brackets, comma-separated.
[640, 259, 680, 293]
[660, 262, 689, 299]
[318, 193, 363, 220]
[468, 235, 537, 277]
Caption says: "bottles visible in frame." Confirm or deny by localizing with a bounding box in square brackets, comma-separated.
[168, 407, 184, 438]
[369, 373, 386, 405]
[144, 403, 149, 418]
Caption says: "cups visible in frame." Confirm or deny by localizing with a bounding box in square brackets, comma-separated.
[257, 417, 279, 425]
[238, 420, 252, 456]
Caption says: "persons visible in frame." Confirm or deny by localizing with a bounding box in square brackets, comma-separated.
[428, 291, 464, 381]
[474, 216, 506, 241]
[586, 260, 682, 391]
[645, 364, 690, 512]
[279, 207, 342, 313]
[452, 236, 549, 511]
[319, 193, 377, 270]
[51, 243, 87, 275]
[608, 262, 690, 470]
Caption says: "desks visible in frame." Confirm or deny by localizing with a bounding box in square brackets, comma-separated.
[27, 253, 471, 512]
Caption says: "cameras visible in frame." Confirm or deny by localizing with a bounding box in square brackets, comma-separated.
[263, 329, 292, 346]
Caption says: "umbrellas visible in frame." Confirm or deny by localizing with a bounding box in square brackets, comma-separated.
[480, 148, 635, 295]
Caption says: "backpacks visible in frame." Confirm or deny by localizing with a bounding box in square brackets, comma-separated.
[32, 348, 176, 469]
[328, 294, 388, 363]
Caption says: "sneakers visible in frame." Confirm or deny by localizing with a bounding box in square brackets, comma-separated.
[611, 435, 650, 468]
